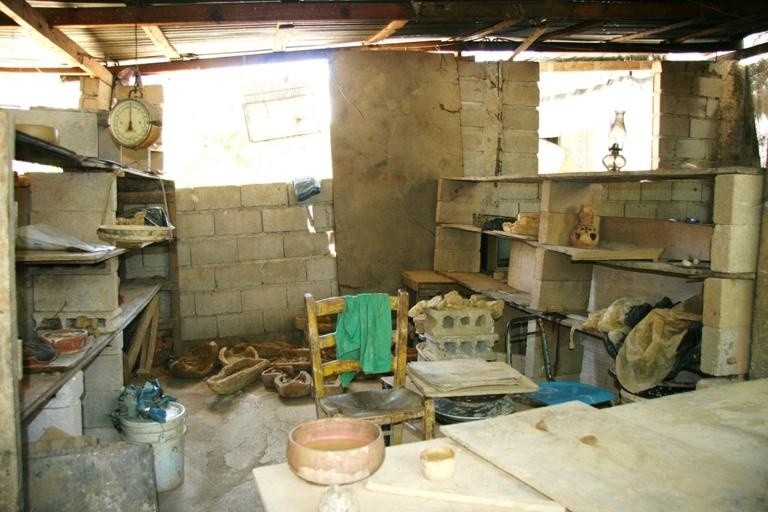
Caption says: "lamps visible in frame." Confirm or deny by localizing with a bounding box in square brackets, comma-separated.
[601, 111, 628, 171]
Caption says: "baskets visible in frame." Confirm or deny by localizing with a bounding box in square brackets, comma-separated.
[96, 224, 176, 248]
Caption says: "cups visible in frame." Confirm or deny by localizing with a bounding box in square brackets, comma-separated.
[419, 446, 458, 480]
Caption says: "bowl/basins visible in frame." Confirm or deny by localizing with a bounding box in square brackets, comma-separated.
[41, 328, 88, 351]
[288, 417, 386, 487]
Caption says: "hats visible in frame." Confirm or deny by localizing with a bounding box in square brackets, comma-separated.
[671, 295, 702, 321]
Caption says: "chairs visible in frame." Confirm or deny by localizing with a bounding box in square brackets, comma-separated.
[505, 314, 617, 405]
[303, 290, 436, 447]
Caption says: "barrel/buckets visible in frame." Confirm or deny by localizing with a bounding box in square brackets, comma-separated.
[121, 400, 188, 496]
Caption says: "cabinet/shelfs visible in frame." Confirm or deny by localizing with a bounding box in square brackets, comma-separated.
[0, 109, 179, 512]
[432, 168, 763, 391]
